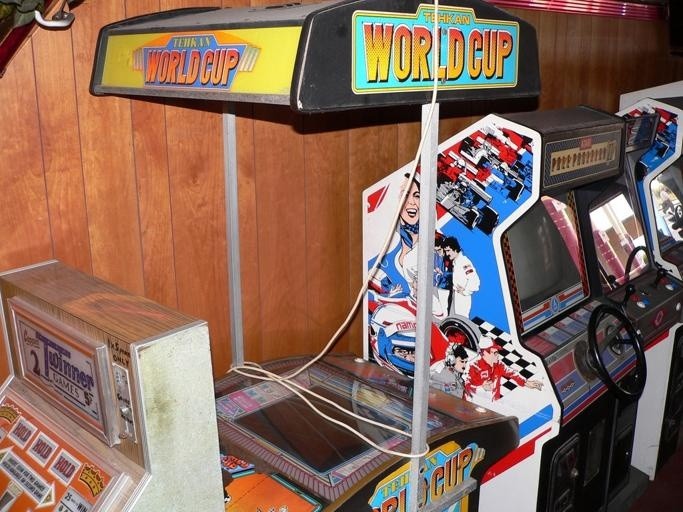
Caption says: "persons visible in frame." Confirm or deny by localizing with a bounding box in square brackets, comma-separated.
[367, 174, 420, 318]
[430, 348, 466, 399]
[467, 339, 543, 405]
[433, 234, 453, 318]
[441, 236, 479, 319]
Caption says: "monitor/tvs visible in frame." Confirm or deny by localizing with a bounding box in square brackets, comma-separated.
[651, 165, 683, 255]
[507, 196, 583, 313]
[589, 190, 652, 295]
[231, 383, 409, 478]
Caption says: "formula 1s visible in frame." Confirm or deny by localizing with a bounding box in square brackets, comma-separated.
[411, 128, 534, 236]
